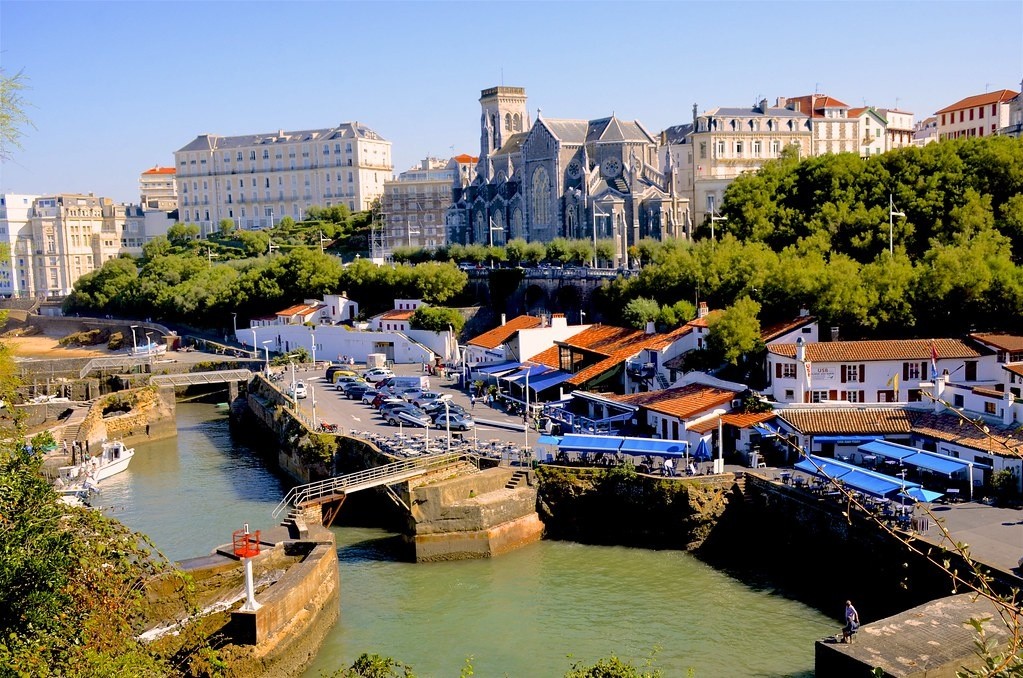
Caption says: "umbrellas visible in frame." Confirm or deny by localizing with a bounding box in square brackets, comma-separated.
[694, 439, 711, 469]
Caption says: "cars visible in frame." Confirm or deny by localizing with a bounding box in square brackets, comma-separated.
[435, 413, 474, 431]
[325, 365, 359, 383]
[386, 407, 432, 428]
[287, 381, 308, 398]
[332, 367, 468, 418]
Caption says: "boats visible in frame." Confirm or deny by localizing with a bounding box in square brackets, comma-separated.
[127, 343, 168, 358]
[51, 439, 134, 511]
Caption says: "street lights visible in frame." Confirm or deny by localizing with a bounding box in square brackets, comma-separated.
[262, 340, 272, 382]
[319, 231, 332, 254]
[268, 238, 280, 254]
[130, 325, 140, 354]
[712, 409, 726, 475]
[488, 216, 504, 268]
[311, 333, 318, 368]
[307, 376, 319, 431]
[231, 312, 242, 344]
[521, 366, 533, 424]
[434, 393, 455, 452]
[592, 200, 610, 268]
[251, 325, 262, 359]
[889, 193, 906, 259]
[710, 203, 728, 243]
[145, 332, 154, 355]
[288, 354, 301, 414]
[207, 246, 219, 267]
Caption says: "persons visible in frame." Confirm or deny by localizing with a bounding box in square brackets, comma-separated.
[63, 439, 90, 463]
[841, 600, 859, 642]
[664, 457, 698, 476]
[469, 394, 541, 431]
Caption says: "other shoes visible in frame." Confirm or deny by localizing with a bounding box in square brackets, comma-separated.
[840, 638, 845, 642]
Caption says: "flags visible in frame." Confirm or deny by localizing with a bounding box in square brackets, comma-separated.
[931, 343, 938, 378]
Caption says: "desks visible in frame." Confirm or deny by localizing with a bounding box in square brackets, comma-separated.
[910, 517, 928, 536]
[362, 431, 370, 440]
[426, 448, 442, 453]
[794, 478, 804, 488]
[511, 449, 520, 454]
[478, 448, 492, 456]
[810, 486, 820, 494]
[480, 443, 490, 448]
[521, 445, 532, 453]
[394, 433, 403, 439]
[875, 498, 891, 510]
[370, 434, 380, 443]
[506, 441, 517, 447]
[494, 445, 506, 452]
[491, 450, 501, 459]
[894, 504, 912, 517]
[780, 472, 791, 483]
[488, 439, 500, 445]
[377, 438, 387, 449]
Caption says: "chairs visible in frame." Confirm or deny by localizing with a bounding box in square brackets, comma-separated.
[772, 453, 961, 531]
[348, 428, 680, 477]
[842, 619, 860, 644]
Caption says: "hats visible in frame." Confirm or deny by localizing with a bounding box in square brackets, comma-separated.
[846, 616, 851, 620]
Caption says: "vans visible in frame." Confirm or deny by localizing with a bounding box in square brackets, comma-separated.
[381, 376, 430, 392]
